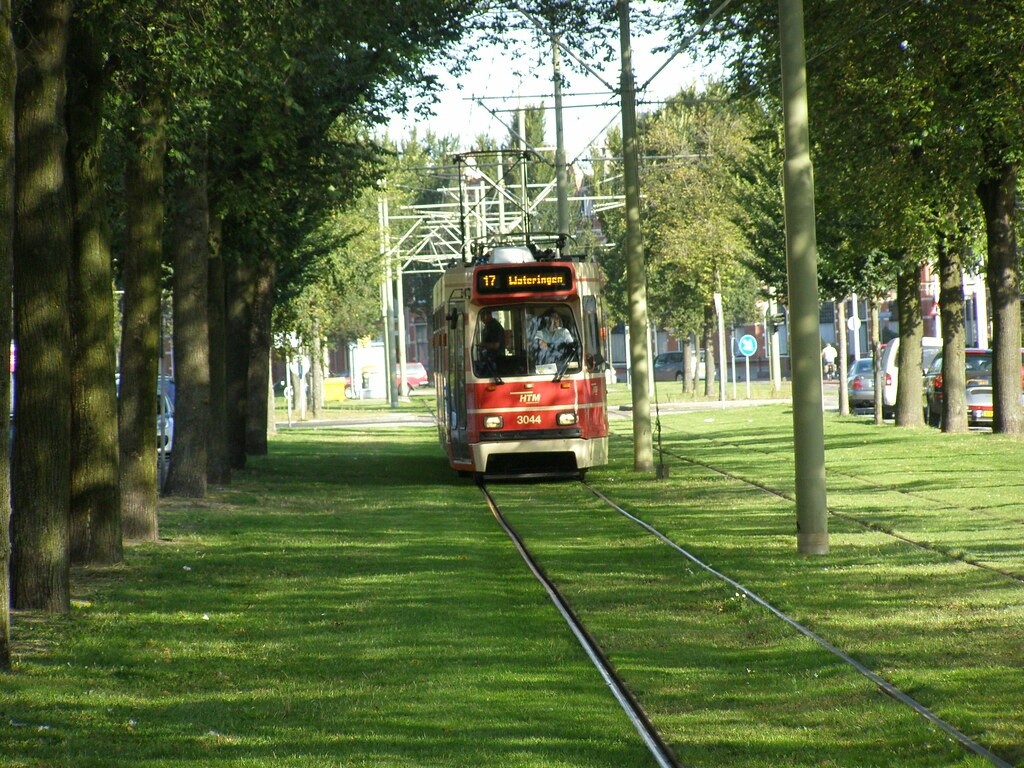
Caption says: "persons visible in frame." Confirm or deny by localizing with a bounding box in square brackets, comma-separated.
[822, 343, 838, 373]
[477, 309, 505, 359]
[534, 311, 574, 350]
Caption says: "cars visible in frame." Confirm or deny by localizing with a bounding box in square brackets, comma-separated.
[922, 348, 994, 431]
[345, 362, 427, 399]
[1021, 347, 1024, 397]
[272, 375, 312, 397]
[823, 362, 837, 381]
[156, 378, 175, 405]
[839, 358, 893, 417]
[115, 379, 175, 455]
[880, 343, 887, 354]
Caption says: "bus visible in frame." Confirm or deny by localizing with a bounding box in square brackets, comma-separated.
[431, 233, 608, 484]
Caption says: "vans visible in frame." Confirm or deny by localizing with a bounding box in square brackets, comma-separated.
[882, 335, 944, 422]
[652, 348, 716, 381]
